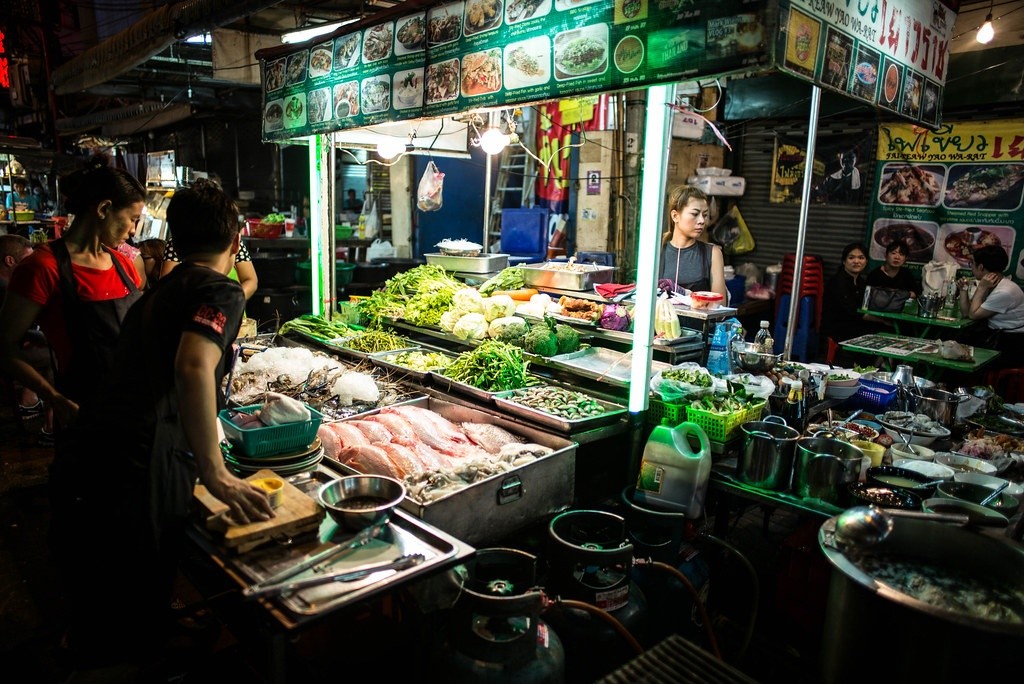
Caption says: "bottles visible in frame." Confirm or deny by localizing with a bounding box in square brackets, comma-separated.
[633, 418, 712, 518]
[731, 328, 746, 364]
[753, 321, 772, 354]
[945, 276, 958, 308]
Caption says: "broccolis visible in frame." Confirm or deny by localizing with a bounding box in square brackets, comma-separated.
[495, 312, 580, 357]
[441, 288, 526, 339]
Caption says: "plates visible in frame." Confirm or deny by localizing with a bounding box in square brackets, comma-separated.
[881, 169, 943, 186]
[875, 415, 951, 438]
[794, 22, 898, 102]
[219, 436, 325, 476]
[266, 0, 645, 130]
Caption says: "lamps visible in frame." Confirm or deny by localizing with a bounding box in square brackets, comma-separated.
[281, 14, 364, 44]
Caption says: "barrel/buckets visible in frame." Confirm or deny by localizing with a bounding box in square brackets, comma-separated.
[917, 295, 941, 319]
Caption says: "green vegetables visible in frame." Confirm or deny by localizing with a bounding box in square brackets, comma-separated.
[561, 37, 605, 72]
[965, 385, 1022, 430]
[358, 263, 470, 326]
[477, 262, 526, 297]
[279, 315, 451, 372]
[662, 368, 767, 415]
[404, 72, 415, 87]
[618, 46, 641, 60]
[777, 363, 876, 380]
[260, 213, 285, 223]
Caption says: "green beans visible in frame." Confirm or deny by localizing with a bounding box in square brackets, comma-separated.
[438, 338, 543, 392]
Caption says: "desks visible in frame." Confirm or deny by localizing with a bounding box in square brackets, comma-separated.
[843, 332, 1001, 380]
[242, 237, 374, 266]
[740, 293, 775, 338]
[856, 308, 973, 337]
[723, 275, 747, 308]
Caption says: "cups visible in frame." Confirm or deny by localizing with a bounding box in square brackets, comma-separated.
[285, 219, 295, 237]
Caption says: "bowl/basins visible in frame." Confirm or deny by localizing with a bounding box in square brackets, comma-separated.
[827, 369, 861, 386]
[826, 382, 863, 399]
[336, 225, 353, 237]
[809, 409, 1024, 533]
[732, 350, 779, 373]
[874, 222, 935, 260]
[252, 252, 429, 286]
[691, 291, 724, 311]
[318, 474, 407, 530]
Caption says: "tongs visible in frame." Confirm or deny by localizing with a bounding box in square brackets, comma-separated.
[240, 521, 428, 601]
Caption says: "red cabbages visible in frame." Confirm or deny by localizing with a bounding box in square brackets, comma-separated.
[601, 304, 633, 331]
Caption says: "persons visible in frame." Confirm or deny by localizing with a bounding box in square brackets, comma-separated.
[663, 183, 728, 334]
[343, 188, 365, 213]
[1, 165, 278, 684]
[818, 239, 1024, 397]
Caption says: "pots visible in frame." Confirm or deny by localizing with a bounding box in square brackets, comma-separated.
[902, 385, 973, 426]
[943, 226, 1002, 270]
[791, 429, 863, 503]
[735, 415, 800, 489]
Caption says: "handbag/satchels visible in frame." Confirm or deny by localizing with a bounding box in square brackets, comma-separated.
[921, 261, 960, 297]
[868, 287, 911, 314]
[358, 199, 381, 242]
[416, 161, 445, 212]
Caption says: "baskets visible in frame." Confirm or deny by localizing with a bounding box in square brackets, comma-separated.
[646, 397, 689, 426]
[685, 401, 765, 443]
[218, 402, 325, 458]
[856, 379, 898, 411]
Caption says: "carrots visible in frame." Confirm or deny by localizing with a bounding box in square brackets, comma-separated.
[493, 289, 539, 307]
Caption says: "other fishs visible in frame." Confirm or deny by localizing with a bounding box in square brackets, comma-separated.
[317, 403, 526, 481]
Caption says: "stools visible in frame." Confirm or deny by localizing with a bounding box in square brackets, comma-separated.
[771, 253, 840, 363]
[986, 366, 1024, 394]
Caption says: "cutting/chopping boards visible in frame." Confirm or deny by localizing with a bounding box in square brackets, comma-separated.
[193, 469, 323, 550]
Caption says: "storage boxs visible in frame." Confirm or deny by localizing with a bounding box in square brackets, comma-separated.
[317, 386, 580, 548]
[500, 205, 550, 267]
